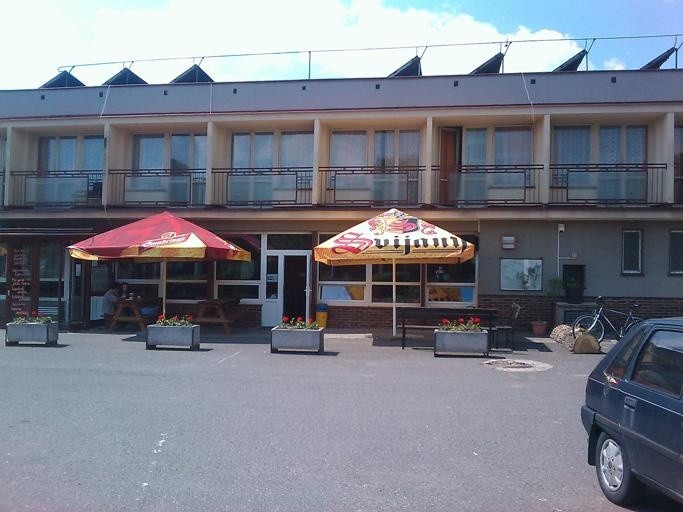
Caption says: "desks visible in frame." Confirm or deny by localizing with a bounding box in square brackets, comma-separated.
[196, 298, 239, 335]
[108, 296, 162, 332]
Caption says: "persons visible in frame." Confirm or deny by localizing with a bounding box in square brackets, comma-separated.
[436, 267, 452, 284]
[101, 280, 131, 330]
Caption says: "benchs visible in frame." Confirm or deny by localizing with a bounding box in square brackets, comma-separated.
[396, 307, 498, 352]
[223, 309, 250, 334]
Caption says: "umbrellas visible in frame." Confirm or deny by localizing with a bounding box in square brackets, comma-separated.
[313, 206, 475, 335]
[66, 210, 251, 318]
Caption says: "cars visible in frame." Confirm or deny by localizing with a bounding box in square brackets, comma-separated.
[579, 316, 682, 507]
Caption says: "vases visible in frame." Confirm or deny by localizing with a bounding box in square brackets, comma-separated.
[270, 325, 324, 354]
[433, 328, 489, 357]
[146, 324, 201, 352]
[5, 321, 58, 346]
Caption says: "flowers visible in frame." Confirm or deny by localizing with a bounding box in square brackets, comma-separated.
[277, 315, 319, 330]
[12, 310, 52, 326]
[154, 313, 193, 327]
[436, 316, 483, 332]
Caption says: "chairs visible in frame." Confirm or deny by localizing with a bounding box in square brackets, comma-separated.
[496, 301, 521, 348]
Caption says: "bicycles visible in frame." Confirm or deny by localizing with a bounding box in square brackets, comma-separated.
[572, 296, 644, 343]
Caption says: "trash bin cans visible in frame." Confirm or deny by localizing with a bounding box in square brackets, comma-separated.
[316, 303, 329, 328]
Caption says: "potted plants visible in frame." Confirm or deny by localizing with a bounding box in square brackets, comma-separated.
[515, 262, 586, 335]
